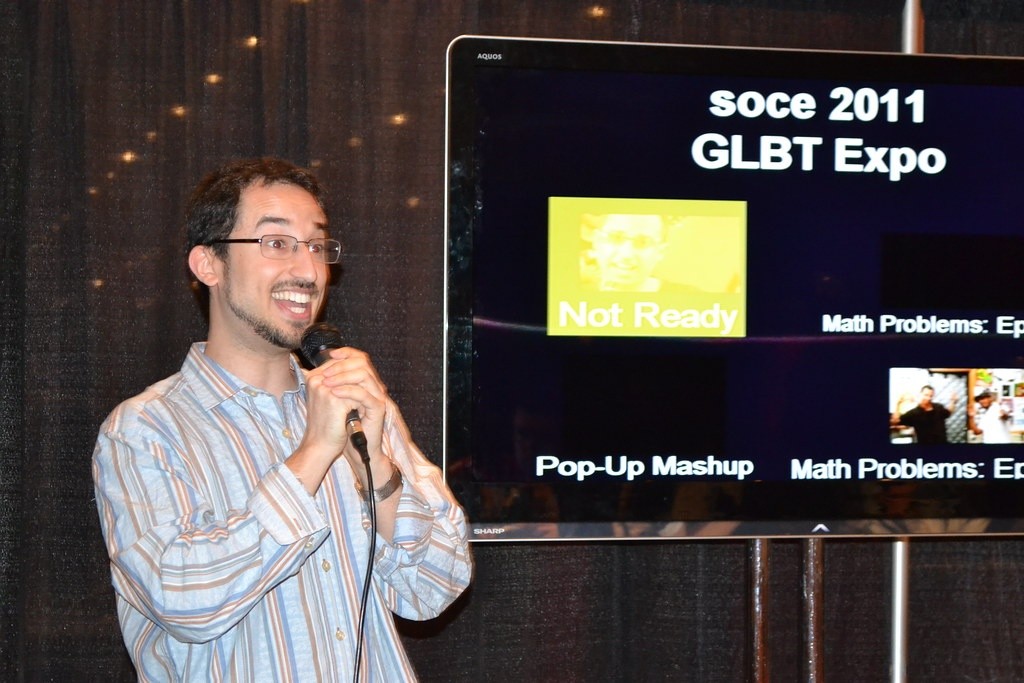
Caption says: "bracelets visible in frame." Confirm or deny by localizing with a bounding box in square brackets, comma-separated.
[355, 460, 403, 502]
[969, 413, 974, 416]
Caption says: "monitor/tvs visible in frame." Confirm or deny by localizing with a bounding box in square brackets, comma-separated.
[444, 33, 1023, 544]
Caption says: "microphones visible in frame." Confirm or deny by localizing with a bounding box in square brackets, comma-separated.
[300, 323, 370, 465]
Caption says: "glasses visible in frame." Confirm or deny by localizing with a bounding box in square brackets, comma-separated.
[201, 234, 342, 264]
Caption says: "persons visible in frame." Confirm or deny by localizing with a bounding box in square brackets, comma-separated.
[967, 388, 1011, 443]
[891, 386, 960, 443]
[93, 157, 473, 683]
